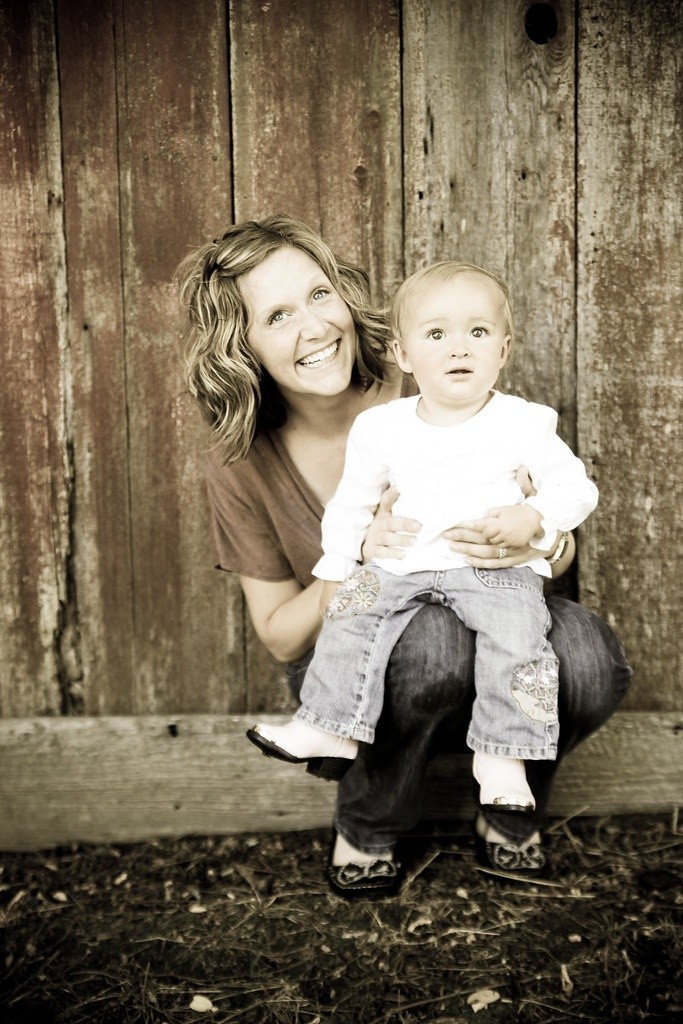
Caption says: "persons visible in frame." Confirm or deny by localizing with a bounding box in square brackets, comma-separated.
[246, 259, 599, 809]
[171, 215, 633, 907]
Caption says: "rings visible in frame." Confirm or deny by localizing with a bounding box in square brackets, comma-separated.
[498, 548, 508, 559]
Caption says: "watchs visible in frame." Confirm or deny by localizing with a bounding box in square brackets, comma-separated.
[546, 532, 569, 565]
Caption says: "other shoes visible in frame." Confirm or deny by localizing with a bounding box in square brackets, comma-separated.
[326, 825, 404, 899]
[473, 811, 548, 878]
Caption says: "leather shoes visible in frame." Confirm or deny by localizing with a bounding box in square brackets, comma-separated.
[472, 752, 536, 813]
[246, 720, 358, 782]
